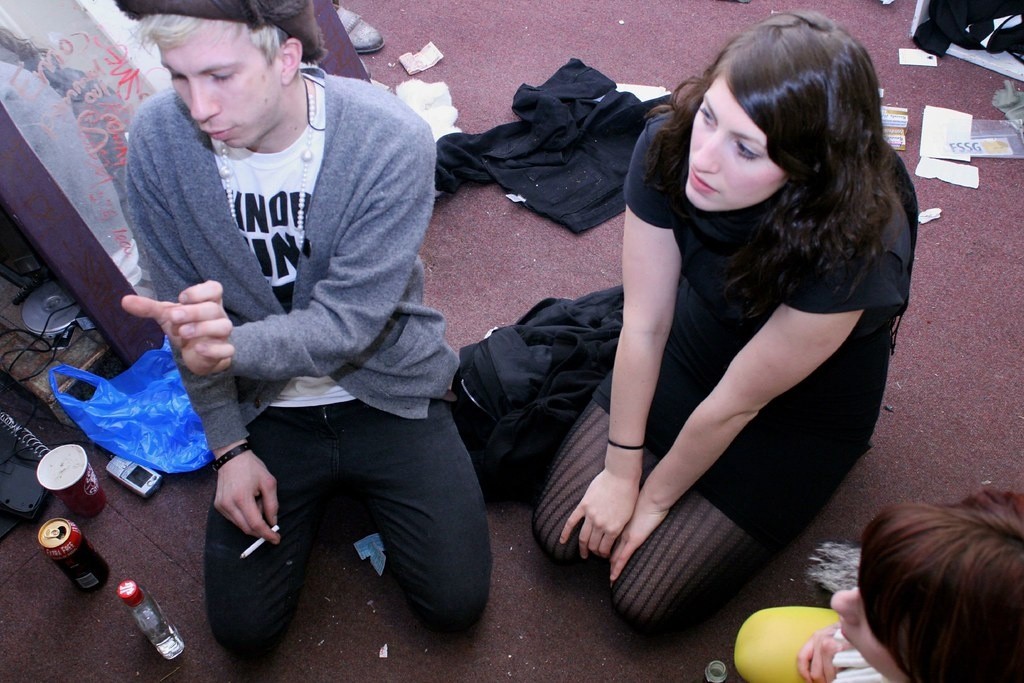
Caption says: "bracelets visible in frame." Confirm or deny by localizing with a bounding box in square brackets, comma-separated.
[211, 442, 253, 472]
[605, 437, 646, 453]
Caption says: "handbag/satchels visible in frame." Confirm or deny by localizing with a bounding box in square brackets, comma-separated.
[47, 344, 223, 474]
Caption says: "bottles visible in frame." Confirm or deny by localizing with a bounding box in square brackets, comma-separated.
[117, 580, 184, 659]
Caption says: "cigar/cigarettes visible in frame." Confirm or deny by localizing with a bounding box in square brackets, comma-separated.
[238, 525, 279, 560]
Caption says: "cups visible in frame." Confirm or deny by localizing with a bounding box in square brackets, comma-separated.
[36, 445, 105, 517]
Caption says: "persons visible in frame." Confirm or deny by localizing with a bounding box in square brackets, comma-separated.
[734, 483, 1024, 683]
[113, 0, 493, 655]
[532, 7, 918, 635]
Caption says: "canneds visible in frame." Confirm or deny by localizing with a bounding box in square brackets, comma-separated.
[37, 518, 109, 593]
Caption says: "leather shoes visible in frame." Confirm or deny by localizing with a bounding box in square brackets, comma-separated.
[336, 6, 383, 52]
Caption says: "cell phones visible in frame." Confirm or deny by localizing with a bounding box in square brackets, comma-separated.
[106, 457, 161, 498]
